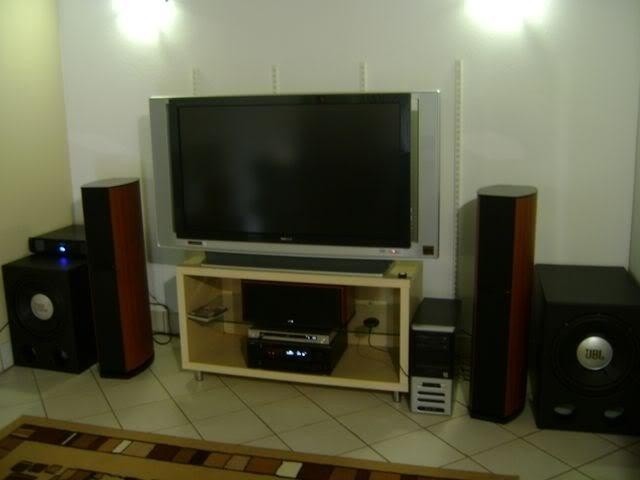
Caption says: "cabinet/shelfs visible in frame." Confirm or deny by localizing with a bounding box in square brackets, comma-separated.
[174, 253, 423, 405]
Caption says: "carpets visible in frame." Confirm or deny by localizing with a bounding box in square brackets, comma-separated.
[0, 414, 520, 480]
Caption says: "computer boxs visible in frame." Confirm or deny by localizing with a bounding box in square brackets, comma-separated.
[410, 298, 461, 416]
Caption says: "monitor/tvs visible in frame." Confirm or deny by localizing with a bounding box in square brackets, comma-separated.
[149, 89, 440, 277]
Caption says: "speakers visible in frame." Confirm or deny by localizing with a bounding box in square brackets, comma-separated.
[468, 185, 538, 424]
[530, 264, 640, 434]
[82, 178, 154, 379]
[1, 253, 98, 375]
